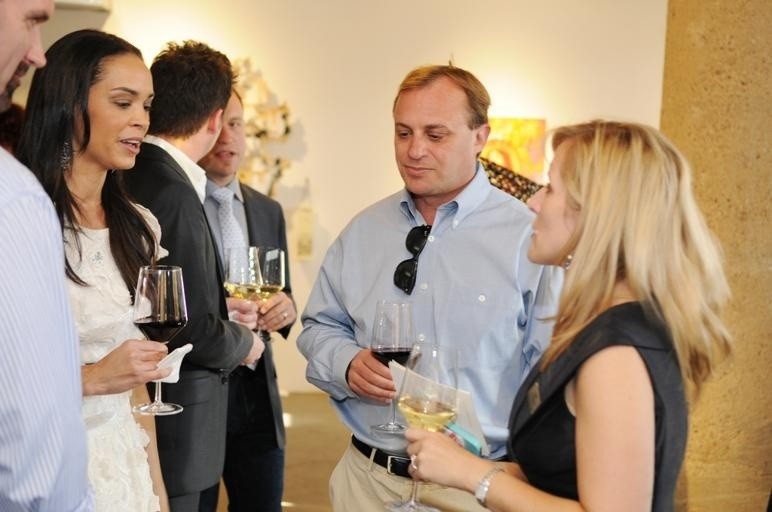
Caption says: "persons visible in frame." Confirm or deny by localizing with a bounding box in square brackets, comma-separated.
[14, 26, 171, 512]
[296, 60, 561, 512]
[121, 39, 267, 512]
[197, 86, 298, 512]
[404, 117, 735, 512]
[0, 0, 100, 512]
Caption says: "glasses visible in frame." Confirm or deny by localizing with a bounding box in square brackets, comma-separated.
[394, 224, 435, 294]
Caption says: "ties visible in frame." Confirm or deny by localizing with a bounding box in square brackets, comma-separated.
[211, 188, 248, 285]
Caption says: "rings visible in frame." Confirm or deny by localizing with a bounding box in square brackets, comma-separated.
[280, 311, 289, 322]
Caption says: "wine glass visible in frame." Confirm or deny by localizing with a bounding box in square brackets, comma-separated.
[223, 245, 286, 336]
[385, 341, 458, 512]
[131, 264, 188, 416]
[370, 300, 417, 435]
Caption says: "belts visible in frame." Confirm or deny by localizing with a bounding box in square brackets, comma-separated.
[351, 434, 410, 479]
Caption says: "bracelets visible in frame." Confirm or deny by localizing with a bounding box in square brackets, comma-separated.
[475, 466, 503, 508]
